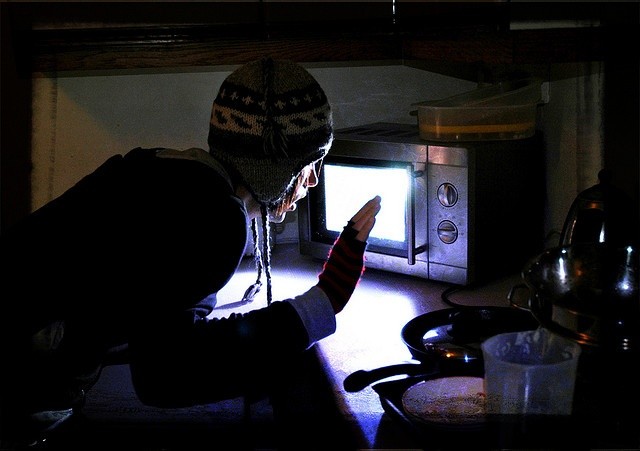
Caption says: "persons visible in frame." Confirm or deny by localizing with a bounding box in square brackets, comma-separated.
[1, 57, 381, 446]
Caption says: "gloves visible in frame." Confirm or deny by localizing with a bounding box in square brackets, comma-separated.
[317, 222, 368, 314]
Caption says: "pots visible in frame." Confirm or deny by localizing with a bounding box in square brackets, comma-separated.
[506, 245, 640, 355]
[344, 305, 540, 393]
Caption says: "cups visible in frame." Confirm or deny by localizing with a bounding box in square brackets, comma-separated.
[481, 327, 581, 417]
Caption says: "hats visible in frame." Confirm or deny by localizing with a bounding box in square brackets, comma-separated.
[208, 54, 334, 203]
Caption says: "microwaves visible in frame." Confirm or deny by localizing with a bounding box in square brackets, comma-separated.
[297, 122, 540, 286]
